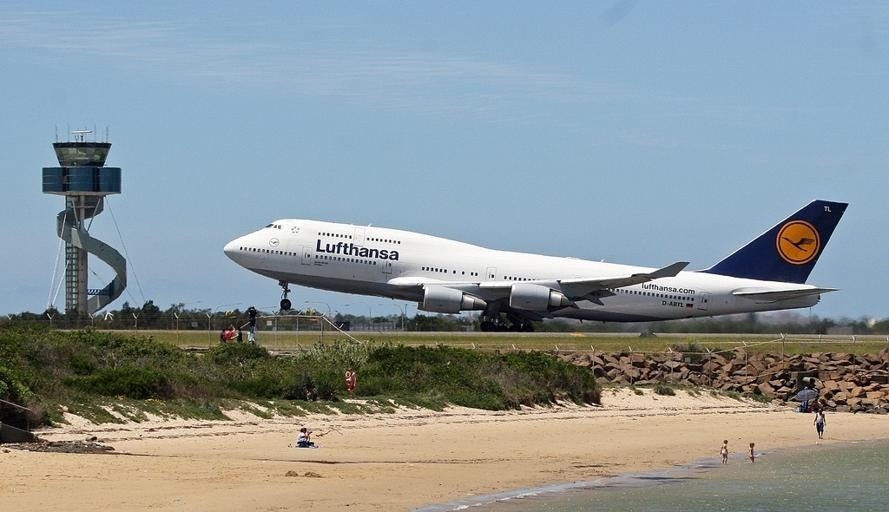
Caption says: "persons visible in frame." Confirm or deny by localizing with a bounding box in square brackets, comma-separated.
[813, 408, 826, 439]
[296, 427, 319, 448]
[344, 367, 357, 399]
[720, 439, 729, 464]
[220, 307, 259, 345]
[794, 385, 823, 413]
[748, 442, 754, 464]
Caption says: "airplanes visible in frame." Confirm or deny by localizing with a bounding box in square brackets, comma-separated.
[222, 199, 848, 332]
